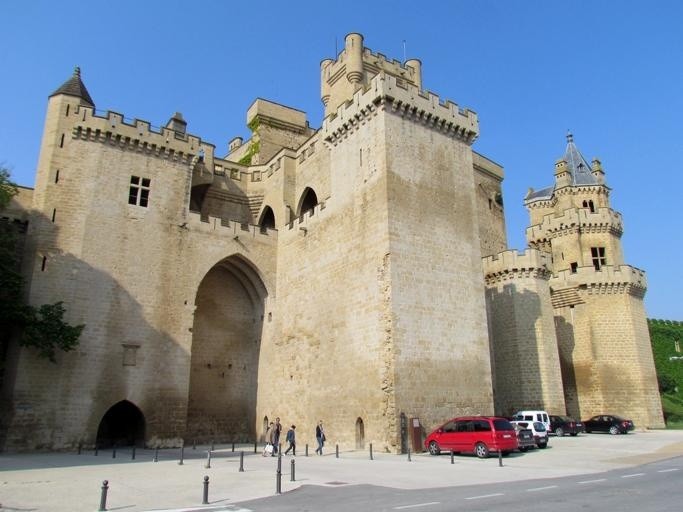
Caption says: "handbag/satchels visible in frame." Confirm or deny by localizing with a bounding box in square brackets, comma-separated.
[321, 433, 326, 441]
[266, 444, 274, 454]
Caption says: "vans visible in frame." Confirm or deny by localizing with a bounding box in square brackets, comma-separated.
[508, 410, 552, 436]
[423, 416, 518, 458]
[509, 420, 548, 450]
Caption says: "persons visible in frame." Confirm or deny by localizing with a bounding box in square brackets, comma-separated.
[282, 425, 296, 458]
[261, 421, 276, 457]
[314, 419, 326, 455]
[274, 417, 282, 454]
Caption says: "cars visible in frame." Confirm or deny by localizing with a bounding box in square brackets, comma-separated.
[578, 414, 635, 435]
[547, 415, 585, 437]
[509, 423, 535, 451]
